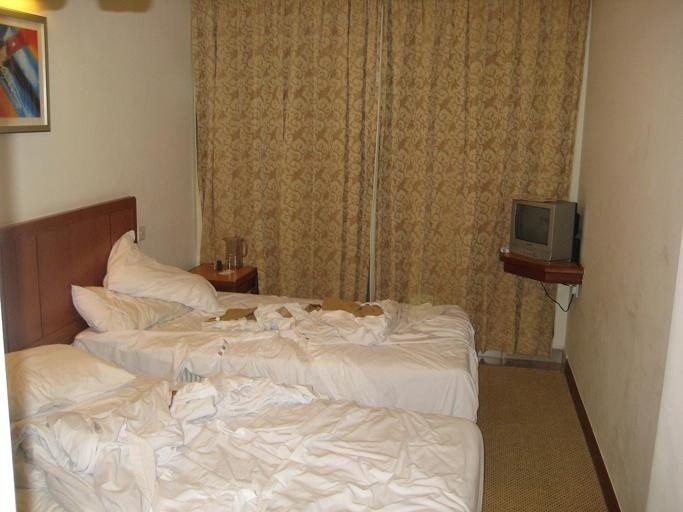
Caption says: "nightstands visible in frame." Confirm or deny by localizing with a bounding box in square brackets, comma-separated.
[186, 262, 258, 294]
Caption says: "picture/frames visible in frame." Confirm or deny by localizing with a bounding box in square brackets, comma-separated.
[0, 6, 51, 134]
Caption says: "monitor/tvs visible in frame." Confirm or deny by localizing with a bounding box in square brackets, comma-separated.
[509, 198, 580, 263]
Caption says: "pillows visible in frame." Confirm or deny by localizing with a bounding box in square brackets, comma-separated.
[71, 284, 190, 333]
[104, 230, 228, 321]
[6, 345, 136, 422]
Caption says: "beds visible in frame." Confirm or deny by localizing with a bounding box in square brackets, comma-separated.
[1, 219, 484, 511]
[35, 196, 480, 424]
